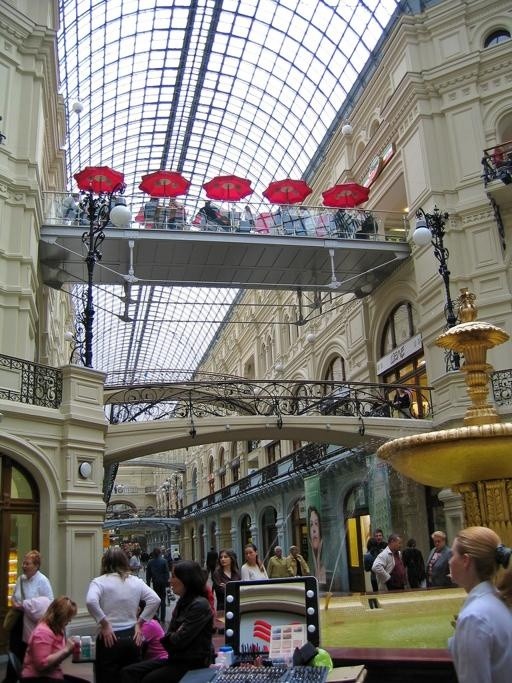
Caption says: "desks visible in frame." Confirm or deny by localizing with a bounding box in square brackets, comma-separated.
[71, 651, 96, 664]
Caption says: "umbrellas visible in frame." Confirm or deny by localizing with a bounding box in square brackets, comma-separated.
[72, 167, 369, 214]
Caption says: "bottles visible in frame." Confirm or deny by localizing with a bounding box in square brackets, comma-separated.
[73, 635, 96, 660]
[214, 652, 227, 672]
[219, 646, 234, 665]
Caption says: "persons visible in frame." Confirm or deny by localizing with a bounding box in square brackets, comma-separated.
[392, 386, 412, 417]
[306, 507, 327, 583]
[58, 192, 379, 240]
[491, 144, 511, 178]
[10, 528, 511, 682]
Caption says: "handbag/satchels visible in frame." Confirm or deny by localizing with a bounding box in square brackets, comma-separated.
[166, 587, 170, 606]
[167, 589, 176, 601]
[3, 608, 20, 632]
[310, 647, 333, 672]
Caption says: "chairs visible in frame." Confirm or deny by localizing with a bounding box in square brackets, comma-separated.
[6, 650, 22, 681]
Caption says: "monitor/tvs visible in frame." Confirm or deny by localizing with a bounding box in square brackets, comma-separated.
[225, 576, 321, 668]
